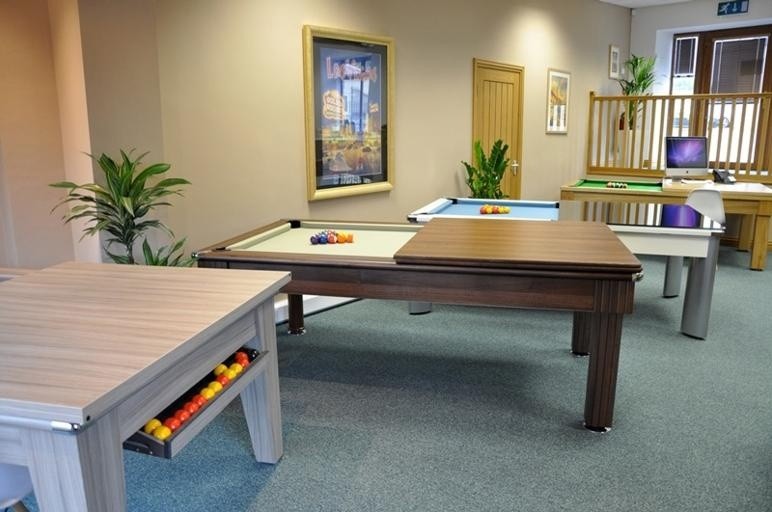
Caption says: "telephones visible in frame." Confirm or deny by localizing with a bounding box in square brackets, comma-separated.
[713, 169, 737, 184]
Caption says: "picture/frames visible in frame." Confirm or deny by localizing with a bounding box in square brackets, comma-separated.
[301, 23, 395, 202]
[546, 66, 571, 136]
[607, 43, 621, 79]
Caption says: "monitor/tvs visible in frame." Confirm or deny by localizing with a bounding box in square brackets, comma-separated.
[660, 203, 704, 228]
[665, 137, 709, 177]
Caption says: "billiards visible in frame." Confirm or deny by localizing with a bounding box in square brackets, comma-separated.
[146, 349, 252, 440]
[310, 228, 353, 244]
[607, 182, 628, 188]
[481, 203, 511, 213]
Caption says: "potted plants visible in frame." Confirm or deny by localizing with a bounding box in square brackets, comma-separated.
[617, 51, 659, 169]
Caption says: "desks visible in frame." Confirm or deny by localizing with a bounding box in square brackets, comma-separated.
[0, 259, 292, 511]
[189, 217, 642, 435]
[405, 194, 727, 341]
[559, 177, 772, 270]
